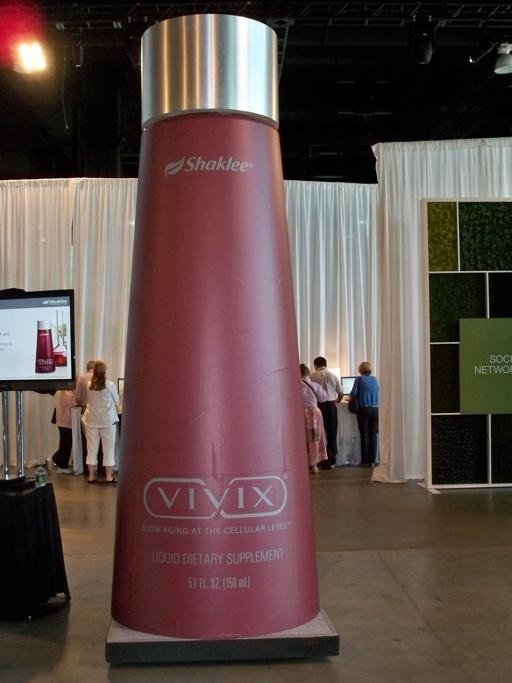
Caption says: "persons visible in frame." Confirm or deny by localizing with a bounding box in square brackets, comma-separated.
[310, 356, 344, 469]
[83, 361, 120, 483]
[350, 362, 380, 468]
[300, 363, 329, 474]
[51, 376, 80, 474]
[76, 360, 101, 475]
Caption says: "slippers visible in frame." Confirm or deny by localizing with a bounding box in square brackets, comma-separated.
[88, 477, 118, 482]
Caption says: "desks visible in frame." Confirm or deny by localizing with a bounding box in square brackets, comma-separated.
[333, 400, 379, 466]
[1, 477, 78, 619]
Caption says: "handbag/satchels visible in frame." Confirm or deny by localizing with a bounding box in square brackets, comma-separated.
[51, 408, 56, 423]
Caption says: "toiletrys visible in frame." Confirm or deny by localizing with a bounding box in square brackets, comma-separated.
[35, 467, 47, 488]
[54, 352, 67, 366]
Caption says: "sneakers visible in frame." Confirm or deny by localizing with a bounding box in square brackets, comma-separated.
[45, 458, 73, 474]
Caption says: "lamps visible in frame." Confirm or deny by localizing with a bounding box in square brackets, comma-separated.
[408, 13, 438, 65]
[492, 29, 511, 74]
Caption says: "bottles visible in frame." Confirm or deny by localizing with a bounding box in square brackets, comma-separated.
[34, 461, 47, 487]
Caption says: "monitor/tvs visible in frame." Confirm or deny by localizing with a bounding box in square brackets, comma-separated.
[341, 377, 357, 394]
[0, 288, 75, 391]
